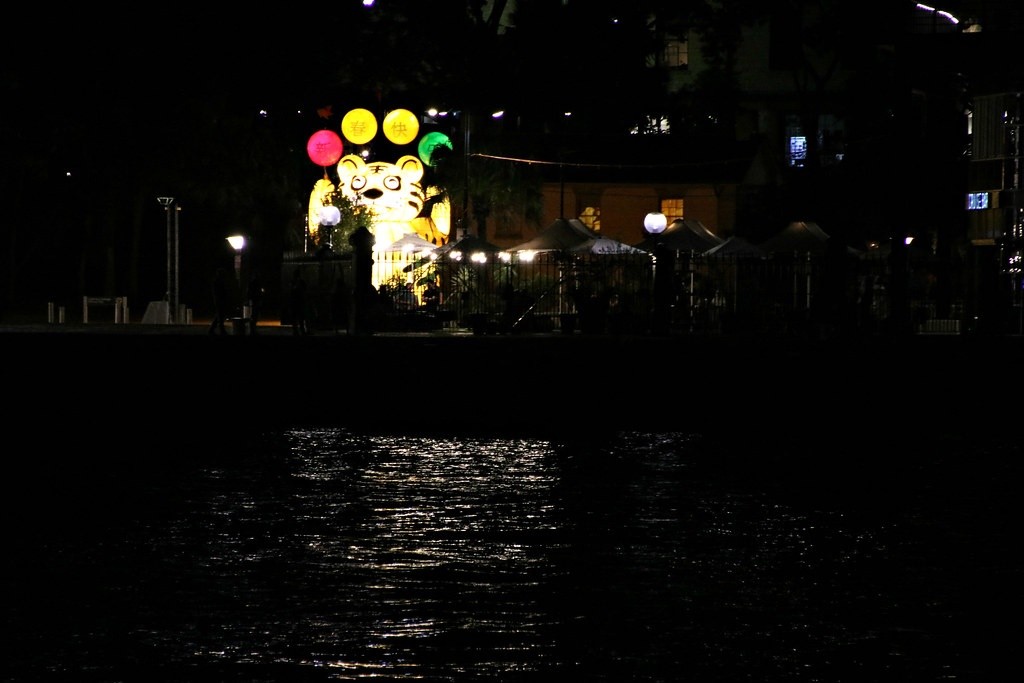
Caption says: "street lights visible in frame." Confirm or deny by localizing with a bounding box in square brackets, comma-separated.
[644, 212, 667, 339]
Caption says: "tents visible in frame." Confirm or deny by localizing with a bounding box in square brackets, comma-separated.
[495, 218, 656, 266]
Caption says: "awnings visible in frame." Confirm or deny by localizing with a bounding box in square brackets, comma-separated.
[633, 219, 862, 268]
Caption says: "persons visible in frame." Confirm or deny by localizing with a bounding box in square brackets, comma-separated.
[421, 278, 441, 331]
[204, 261, 309, 335]
